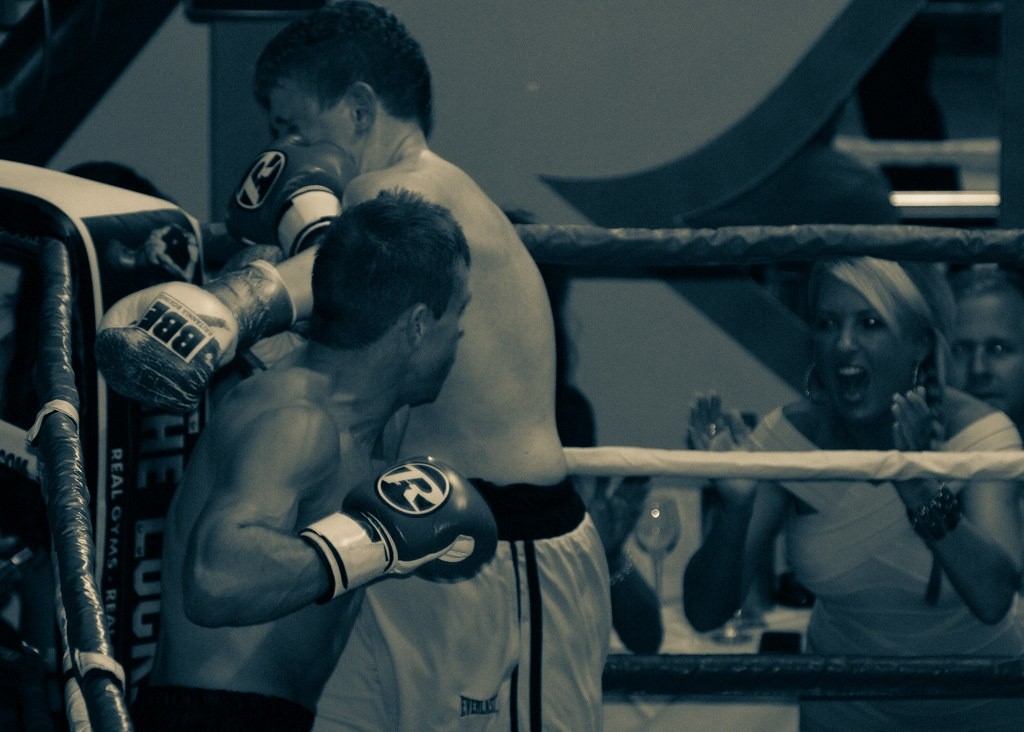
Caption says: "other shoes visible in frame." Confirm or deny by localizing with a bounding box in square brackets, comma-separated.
[604, 545, 664, 656]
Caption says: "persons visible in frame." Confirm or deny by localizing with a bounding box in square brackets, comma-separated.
[0, 1, 664, 732]
[680, 256, 1024, 732]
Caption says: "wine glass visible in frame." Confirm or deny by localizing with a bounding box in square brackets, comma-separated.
[634, 498, 681, 604]
[712, 613, 751, 643]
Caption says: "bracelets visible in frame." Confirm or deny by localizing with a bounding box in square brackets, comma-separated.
[912, 485, 966, 550]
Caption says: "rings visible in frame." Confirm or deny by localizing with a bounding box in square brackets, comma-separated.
[707, 423, 717, 438]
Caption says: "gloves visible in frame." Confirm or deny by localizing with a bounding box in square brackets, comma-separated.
[93, 261, 298, 413]
[300, 453, 500, 605]
[226, 129, 345, 256]
